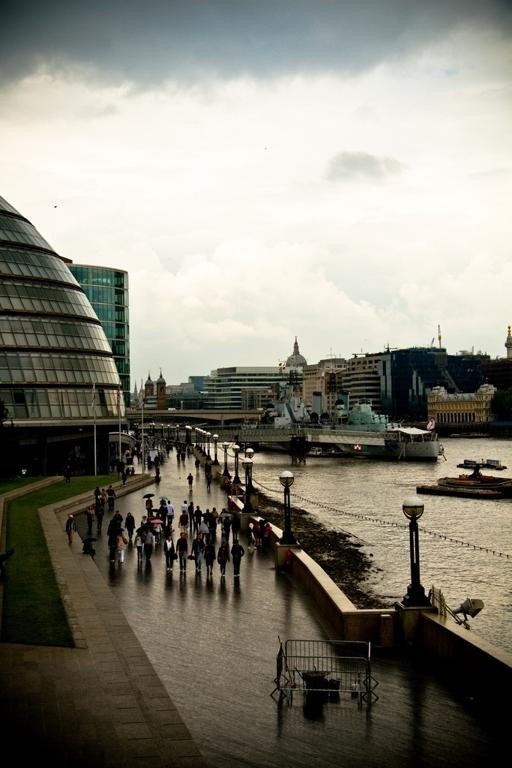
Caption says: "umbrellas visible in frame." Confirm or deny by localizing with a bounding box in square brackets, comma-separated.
[187, 553, 196, 560]
[174, 551, 178, 560]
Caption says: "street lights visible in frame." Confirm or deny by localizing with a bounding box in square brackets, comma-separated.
[241, 457, 256, 513]
[194, 427, 219, 466]
[232, 444, 242, 484]
[278, 470, 297, 545]
[245, 448, 258, 492]
[222, 441, 230, 477]
[400, 497, 431, 607]
[149, 422, 192, 446]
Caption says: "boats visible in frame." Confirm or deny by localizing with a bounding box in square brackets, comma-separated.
[416, 463, 511, 501]
[385, 426, 440, 459]
[457, 456, 507, 471]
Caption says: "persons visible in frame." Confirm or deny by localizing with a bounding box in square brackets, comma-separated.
[217, 541, 230, 576]
[163, 535, 175, 572]
[231, 539, 244, 577]
[66, 514, 77, 545]
[191, 532, 205, 573]
[83, 483, 273, 550]
[203, 540, 216, 575]
[176, 532, 189, 571]
[224, 477, 229, 488]
[64, 466, 72, 484]
[108, 532, 119, 562]
[82, 542, 96, 561]
[143, 528, 155, 560]
[135, 530, 145, 559]
[116, 531, 128, 565]
[110, 435, 213, 491]
